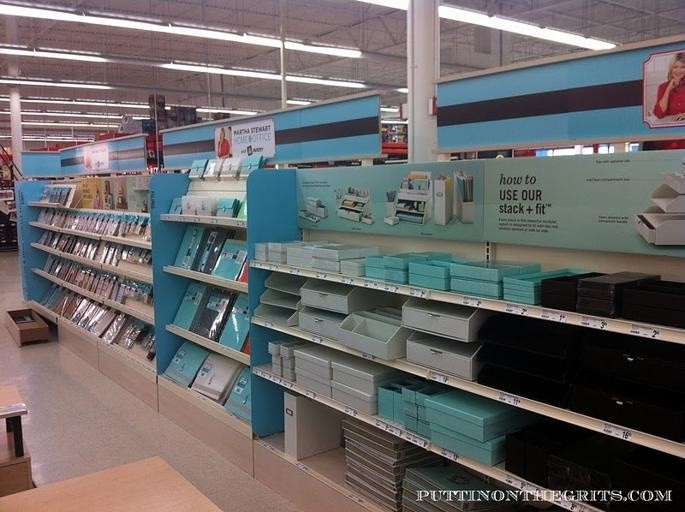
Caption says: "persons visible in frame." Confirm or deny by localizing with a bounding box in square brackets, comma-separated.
[653, 52, 685, 123]
[216, 128, 230, 159]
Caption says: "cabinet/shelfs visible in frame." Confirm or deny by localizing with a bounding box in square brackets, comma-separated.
[29, 167, 684, 512]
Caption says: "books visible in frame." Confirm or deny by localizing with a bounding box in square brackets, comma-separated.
[27, 174, 156, 364]
